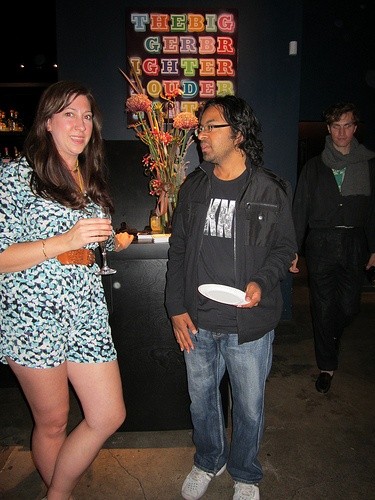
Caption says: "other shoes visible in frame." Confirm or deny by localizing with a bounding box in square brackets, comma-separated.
[315, 371, 334, 393]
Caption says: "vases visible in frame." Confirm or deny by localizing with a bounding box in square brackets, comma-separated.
[137, 225, 171, 243]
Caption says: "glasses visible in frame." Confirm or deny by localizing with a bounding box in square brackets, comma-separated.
[194, 125, 230, 136]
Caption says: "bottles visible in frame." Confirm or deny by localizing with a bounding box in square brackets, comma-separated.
[150, 210, 162, 232]
[0, 109, 23, 131]
[0, 146, 23, 163]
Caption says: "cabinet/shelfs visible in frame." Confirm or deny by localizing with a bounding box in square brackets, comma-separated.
[0, 81, 46, 163]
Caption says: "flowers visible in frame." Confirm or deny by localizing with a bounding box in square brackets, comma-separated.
[120, 57, 208, 227]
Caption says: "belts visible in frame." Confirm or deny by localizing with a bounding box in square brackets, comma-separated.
[56, 250, 96, 267]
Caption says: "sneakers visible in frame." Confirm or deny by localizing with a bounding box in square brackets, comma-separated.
[232, 480, 260, 500]
[181, 461, 227, 500]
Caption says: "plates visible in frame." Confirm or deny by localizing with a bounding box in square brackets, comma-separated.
[198, 284, 250, 306]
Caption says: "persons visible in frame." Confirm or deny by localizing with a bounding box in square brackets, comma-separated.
[286, 102, 375, 393]
[165, 95, 298, 500]
[0, 79, 132, 500]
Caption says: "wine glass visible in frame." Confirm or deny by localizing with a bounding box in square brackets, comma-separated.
[92, 208, 117, 274]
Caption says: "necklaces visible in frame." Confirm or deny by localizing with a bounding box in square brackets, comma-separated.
[67, 164, 78, 174]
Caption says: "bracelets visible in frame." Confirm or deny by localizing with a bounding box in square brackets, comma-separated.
[42, 239, 49, 258]
